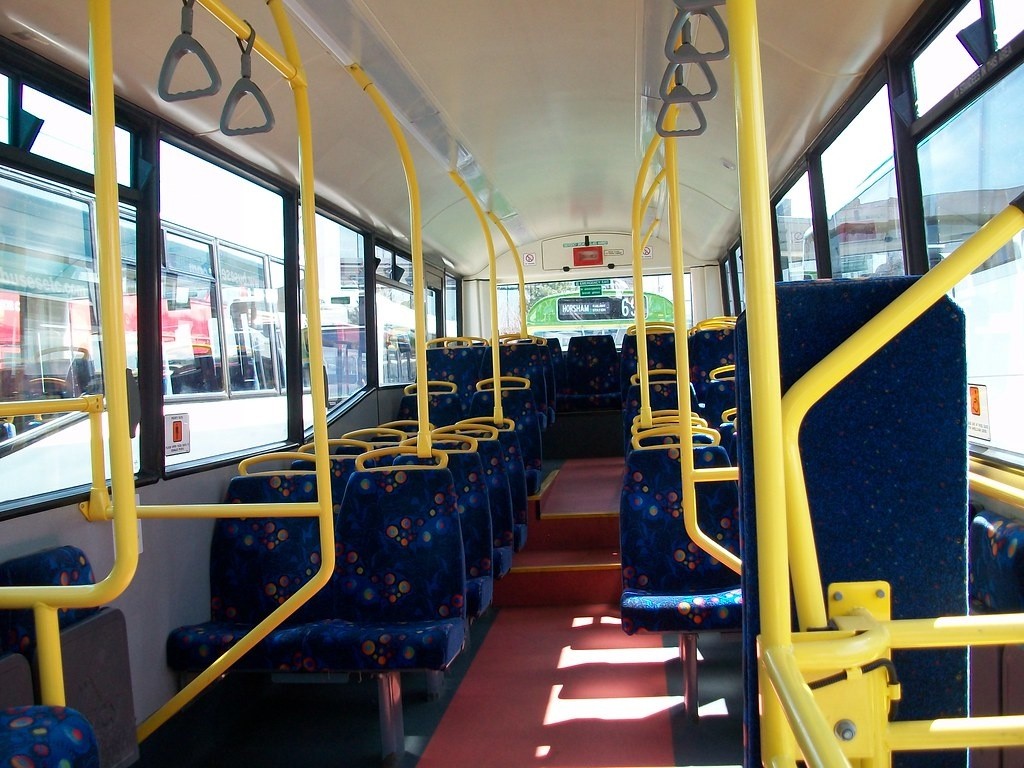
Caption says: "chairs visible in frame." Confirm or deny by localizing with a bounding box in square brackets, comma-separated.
[0, 277, 1024, 768]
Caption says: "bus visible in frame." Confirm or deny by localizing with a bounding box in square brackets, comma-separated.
[1, 161, 456, 507]
[797, 58, 1024, 488]
